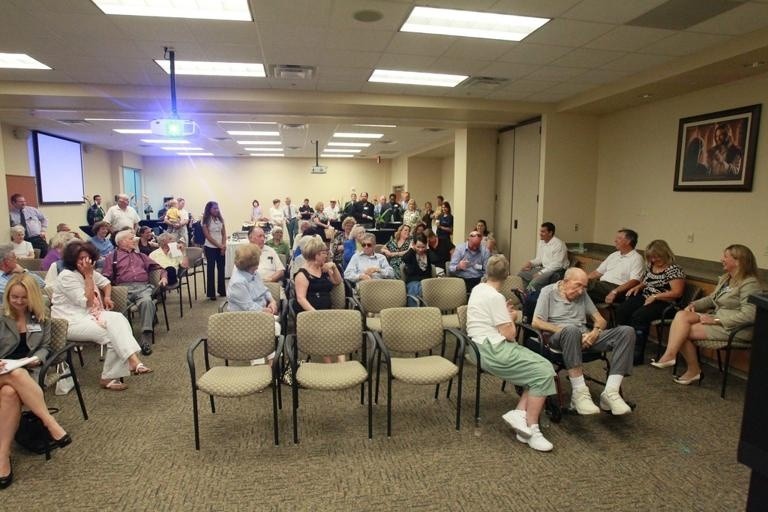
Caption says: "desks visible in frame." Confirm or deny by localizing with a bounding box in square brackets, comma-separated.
[366, 227, 397, 244]
[388, 221, 403, 229]
[224, 238, 250, 279]
[139, 219, 167, 235]
[79, 226, 98, 237]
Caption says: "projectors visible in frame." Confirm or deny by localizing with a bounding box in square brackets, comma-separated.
[311, 166, 326, 174]
[150, 118, 199, 138]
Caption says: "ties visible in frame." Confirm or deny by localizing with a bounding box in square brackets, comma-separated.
[20, 209, 26, 234]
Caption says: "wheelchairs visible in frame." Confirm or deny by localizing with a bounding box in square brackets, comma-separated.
[510, 288, 636, 423]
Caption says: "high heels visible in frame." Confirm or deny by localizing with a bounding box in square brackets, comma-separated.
[650, 358, 676, 368]
[673, 371, 704, 385]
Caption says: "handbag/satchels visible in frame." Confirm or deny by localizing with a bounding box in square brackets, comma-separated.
[15, 410, 58, 454]
[614, 321, 649, 365]
[55, 361, 79, 395]
[279, 360, 306, 388]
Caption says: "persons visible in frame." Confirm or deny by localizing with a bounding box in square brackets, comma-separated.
[582, 229, 686, 383]
[467, 254, 556, 451]
[652, 244, 763, 385]
[226, 192, 496, 385]
[2, 193, 188, 294]
[1, 272, 72, 490]
[531, 267, 636, 415]
[201, 202, 226, 301]
[702, 124, 742, 177]
[517, 222, 570, 311]
[682, 137, 707, 182]
[51, 242, 152, 391]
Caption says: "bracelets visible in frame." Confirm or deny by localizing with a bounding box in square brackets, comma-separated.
[714, 317, 720, 325]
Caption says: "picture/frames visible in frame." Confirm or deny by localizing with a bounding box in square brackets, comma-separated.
[673, 103, 761, 192]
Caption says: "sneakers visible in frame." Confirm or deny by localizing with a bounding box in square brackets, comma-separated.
[502, 409, 531, 439]
[516, 424, 553, 451]
[570, 386, 600, 415]
[600, 388, 631, 415]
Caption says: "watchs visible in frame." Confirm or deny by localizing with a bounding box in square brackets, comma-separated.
[593, 327, 603, 334]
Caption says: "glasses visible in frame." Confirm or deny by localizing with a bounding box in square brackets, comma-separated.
[362, 244, 371, 247]
[468, 234, 482, 237]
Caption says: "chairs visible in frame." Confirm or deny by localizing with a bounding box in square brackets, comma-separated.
[218, 256, 524, 367]
[19, 315, 90, 424]
[672, 321, 755, 399]
[650, 283, 703, 362]
[374, 305, 466, 440]
[290, 309, 376, 443]
[186, 310, 285, 447]
[445, 304, 545, 436]
[17, 248, 207, 360]
[593, 303, 619, 330]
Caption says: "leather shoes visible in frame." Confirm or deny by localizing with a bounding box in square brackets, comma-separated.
[0, 456, 12, 489]
[55, 434, 72, 448]
[141, 340, 152, 354]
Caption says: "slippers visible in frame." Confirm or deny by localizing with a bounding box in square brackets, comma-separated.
[130, 363, 151, 375]
[101, 379, 128, 390]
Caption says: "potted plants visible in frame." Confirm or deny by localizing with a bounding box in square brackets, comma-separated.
[373, 207, 393, 229]
